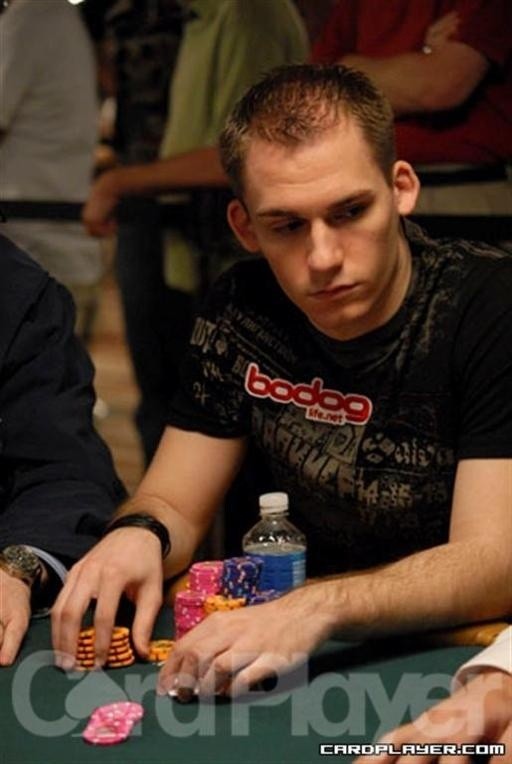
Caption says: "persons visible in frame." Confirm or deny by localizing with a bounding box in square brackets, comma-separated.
[348, 625, 512, 764]
[0, 233, 128, 663]
[0, 0, 512, 287]
[45, 63, 512, 699]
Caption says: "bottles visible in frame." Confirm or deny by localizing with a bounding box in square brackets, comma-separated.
[245, 489, 313, 680]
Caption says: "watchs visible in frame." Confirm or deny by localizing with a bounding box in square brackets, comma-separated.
[0, 543, 43, 599]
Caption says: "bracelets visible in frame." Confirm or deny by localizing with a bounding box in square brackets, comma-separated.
[106, 513, 170, 560]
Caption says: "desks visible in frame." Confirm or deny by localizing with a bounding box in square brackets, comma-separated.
[0, 573, 509, 763]
